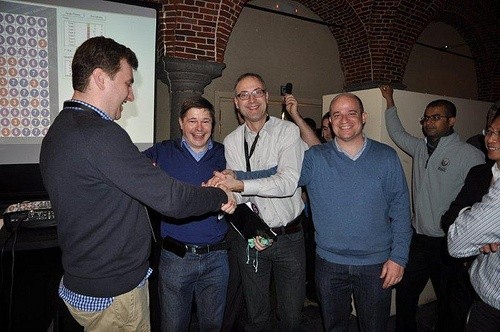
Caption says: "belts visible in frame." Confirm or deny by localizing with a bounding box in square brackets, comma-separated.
[270, 216, 302, 235]
[185, 241, 230, 254]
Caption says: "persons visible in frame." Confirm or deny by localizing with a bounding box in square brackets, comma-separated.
[39, 36, 229, 332]
[439, 101, 499, 332]
[380, 83, 489, 332]
[208, 91, 412, 332]
[206, 73, 304, 332]
[137, 91, 236, 332]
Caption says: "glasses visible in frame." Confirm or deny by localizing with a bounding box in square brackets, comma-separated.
[237, 89, 265, 100]
[482, 129, 500, 137]
[421, 114, 448, 120]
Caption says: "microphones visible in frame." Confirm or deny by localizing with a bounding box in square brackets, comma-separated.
[286, 83, 293, 95]
